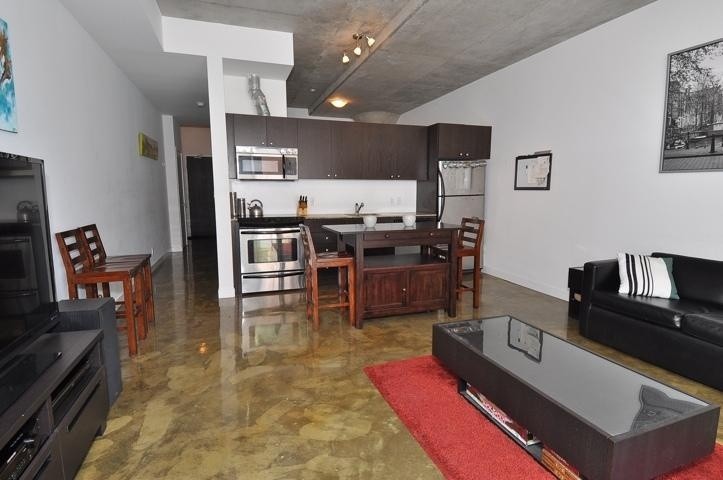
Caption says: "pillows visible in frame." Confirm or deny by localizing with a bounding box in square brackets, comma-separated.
[615, 250, 680, 299]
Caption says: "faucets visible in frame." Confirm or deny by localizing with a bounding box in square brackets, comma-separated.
[355, 203, 365, 214]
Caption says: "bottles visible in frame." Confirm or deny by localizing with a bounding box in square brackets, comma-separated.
[238, 198, 246, 218]
[230, 192, 237, 218]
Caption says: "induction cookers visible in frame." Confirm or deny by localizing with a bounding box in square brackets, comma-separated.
[238, 215, 304, 230]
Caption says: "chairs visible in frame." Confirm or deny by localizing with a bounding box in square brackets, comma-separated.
[77, 223, 153, 331]
[53, 228, 148, 358]
[429, 218, 488, 313]
[296, 223, 356, 331]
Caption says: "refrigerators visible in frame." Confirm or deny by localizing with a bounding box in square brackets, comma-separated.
[438, 160, 485, 274]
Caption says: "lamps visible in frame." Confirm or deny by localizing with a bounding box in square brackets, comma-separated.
[340, 31, 376, 65]
[326, 96, 350, 109]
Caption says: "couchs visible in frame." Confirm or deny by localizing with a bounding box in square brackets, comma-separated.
[578, 249, 722, 391]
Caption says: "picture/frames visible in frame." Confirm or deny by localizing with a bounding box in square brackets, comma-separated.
[657, 36, 722, 175]
[138, 132, 159, 161]
[513, 152, 553, 191]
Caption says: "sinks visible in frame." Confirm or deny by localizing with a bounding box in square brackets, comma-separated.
[345, 213, 379, 218]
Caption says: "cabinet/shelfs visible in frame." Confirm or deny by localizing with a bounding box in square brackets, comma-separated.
[297, 117, 364, 180]
[303, 217, 396, 290]
[225, 112, 298, 181]
[429, 122, 491, 161]
[364, 122, 428, 182]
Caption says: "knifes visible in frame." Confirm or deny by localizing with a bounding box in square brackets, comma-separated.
[299, 195, 307, 203]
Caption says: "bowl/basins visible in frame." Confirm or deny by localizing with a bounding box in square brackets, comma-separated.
[403, 226, 414, 229]
[364, 228, 376, 231]
[353, 111, 400, 125]
[361, 215, 378, 227]
[401, 215, 416, 226]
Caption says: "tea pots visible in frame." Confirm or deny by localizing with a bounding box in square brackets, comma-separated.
[247, 200, 264, 216]
[15, 200, 40, 224]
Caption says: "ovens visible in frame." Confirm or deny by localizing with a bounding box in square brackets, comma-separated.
[241, 294, 308, 360]
[239, 227, 306, 295]
[0, 236, 39, 314]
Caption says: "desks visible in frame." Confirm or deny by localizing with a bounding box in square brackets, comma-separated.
[567, 265, 583, 319]
[318, 220, 475, 331]
[0, 329, 114, 480]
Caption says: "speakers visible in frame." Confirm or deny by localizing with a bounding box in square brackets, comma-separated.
[49, 297, 123, 410]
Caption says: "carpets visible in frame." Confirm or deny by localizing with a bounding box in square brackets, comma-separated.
[361, 353, 722, 479]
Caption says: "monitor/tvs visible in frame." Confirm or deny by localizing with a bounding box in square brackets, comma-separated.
[0, 152, 61, 416]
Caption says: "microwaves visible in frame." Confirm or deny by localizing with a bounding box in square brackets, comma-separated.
[235, 145, 299, 180]
